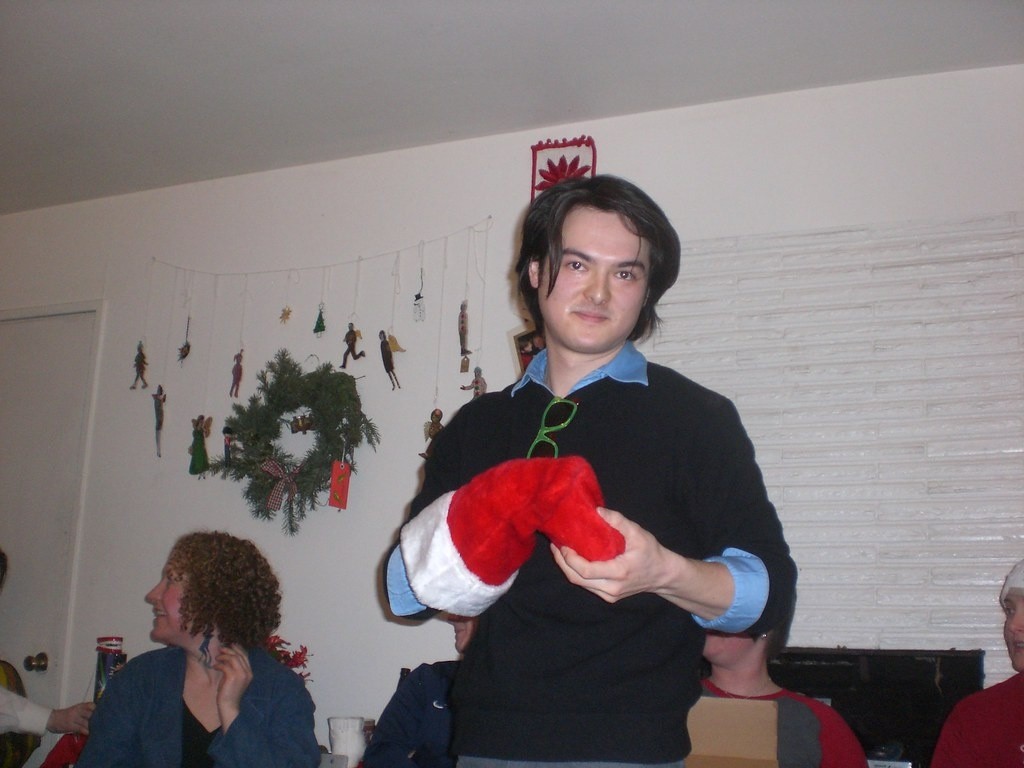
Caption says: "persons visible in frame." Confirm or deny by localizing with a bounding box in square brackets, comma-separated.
[0, 547, 96, 736]
[374, 173, 799, 768]
[362, 614, 480, 768]
[684, 587, 869, 768]
[931, 559, 1024, 768]
[73, 531, 322, 768]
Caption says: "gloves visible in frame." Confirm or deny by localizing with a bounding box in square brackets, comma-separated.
[400, 456, 626, 617]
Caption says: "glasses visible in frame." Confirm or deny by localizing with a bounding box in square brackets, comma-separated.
[526, 396, 577, 457]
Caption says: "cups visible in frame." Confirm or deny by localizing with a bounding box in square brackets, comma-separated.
[327, 716, 364, 768]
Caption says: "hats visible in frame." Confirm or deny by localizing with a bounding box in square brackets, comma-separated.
[1000, 559, 1024, 608]
